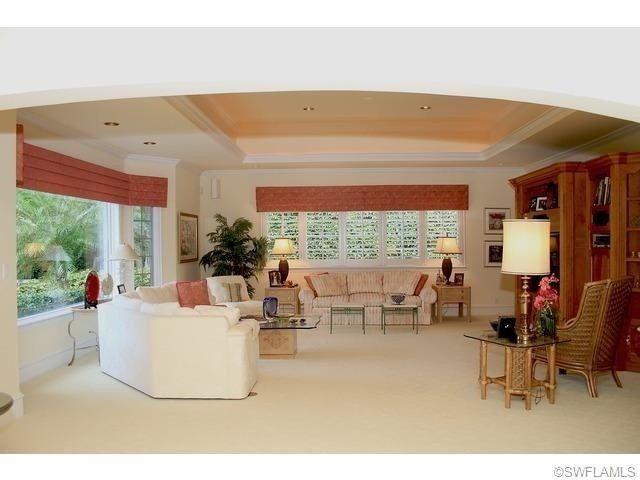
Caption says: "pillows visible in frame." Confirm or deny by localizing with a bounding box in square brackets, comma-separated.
[222, 282, 243, 302]
[176, 279, 211, 310]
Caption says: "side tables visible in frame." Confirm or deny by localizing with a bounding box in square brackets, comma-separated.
[265, 286, 300, 314]
[330, 303, 366, 334]
[239, 314, 322, 360]
[464, 331, 571, 410]
[432, 283, 471, 323]
[382, 302, 419, 334]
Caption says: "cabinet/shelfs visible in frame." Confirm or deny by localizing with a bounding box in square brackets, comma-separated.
[508, 151, 638, 373]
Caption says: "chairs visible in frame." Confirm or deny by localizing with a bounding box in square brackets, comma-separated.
[530, 276, 634, 398]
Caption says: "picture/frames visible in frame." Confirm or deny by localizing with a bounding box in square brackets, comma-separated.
[483, 207, 511, 267]
[179, 212, 199, 264]
[454, 272, 464, 286]
[268, 272, 283, 287]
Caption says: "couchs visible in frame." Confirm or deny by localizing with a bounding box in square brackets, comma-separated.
[97, 274, 264, 402]
[299, 273, 437, 327]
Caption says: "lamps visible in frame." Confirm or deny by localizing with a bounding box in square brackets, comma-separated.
[501, 212, 551, 341]
[270, 236, 297, 284]
[433, 231, 462, 284]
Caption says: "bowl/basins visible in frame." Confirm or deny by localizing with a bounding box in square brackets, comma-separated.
[490, 321, 498, 333]
[392, 295, 405, 305]
